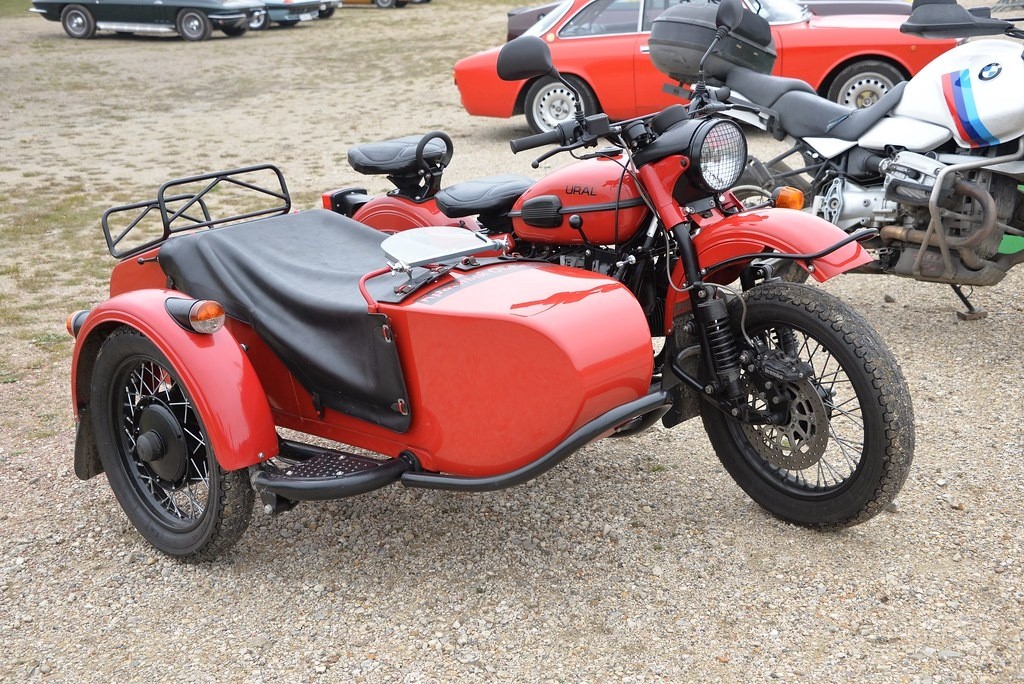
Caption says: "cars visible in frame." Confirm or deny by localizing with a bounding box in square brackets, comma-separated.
[453, 0, 998, 133]
[29, 0, 432, 42]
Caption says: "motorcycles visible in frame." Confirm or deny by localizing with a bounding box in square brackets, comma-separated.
[66, 1, 915, 564]
[649, 0, 1024, 321]
